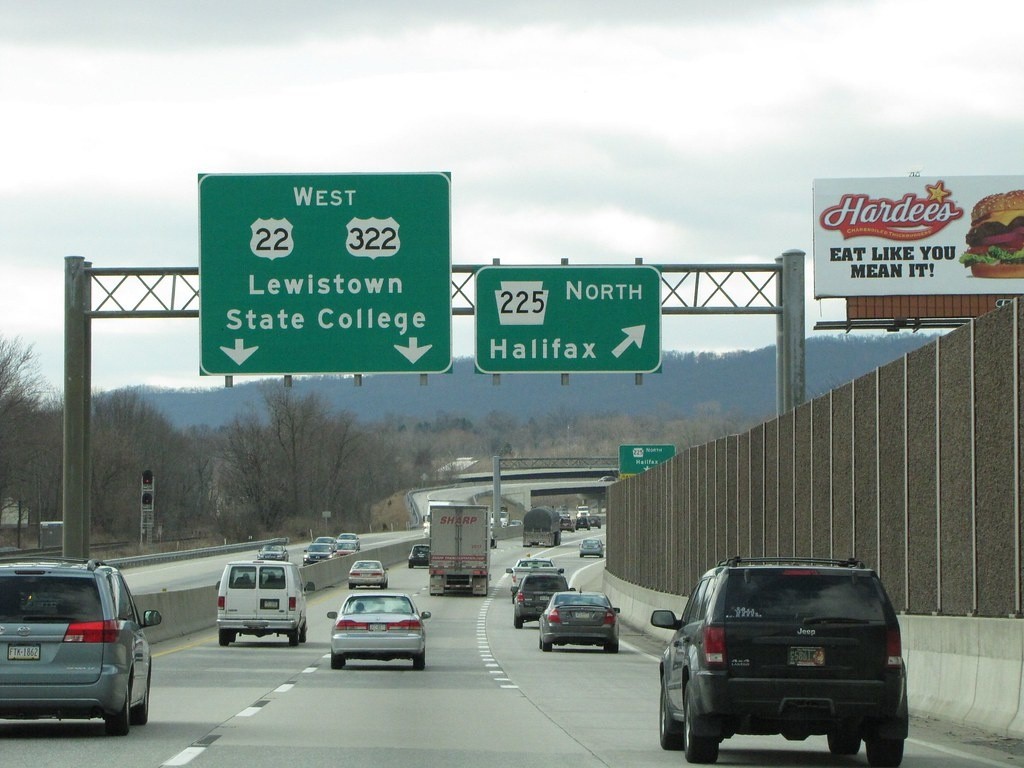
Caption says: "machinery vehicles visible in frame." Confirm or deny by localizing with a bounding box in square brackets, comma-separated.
[522, 508, 563, 548]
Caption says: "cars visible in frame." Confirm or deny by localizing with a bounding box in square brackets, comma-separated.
[311, 536, 335, 544]
[510, 574, 577, 629]
[557, 505, 601, 533]
[348, 560, 389, 589]
[598, 475, 617, 482]
[303, 543, 337, 566]
[335, 539, 361, 556]
[336, 533, 360, 542]
[490, 512, 522, 548]
[408, 544, 431, 569]
[326, 592, 432, 673]
[579, 539, 605, 558]
[535, 591, 621, 654]
[257, 544, 289, 562]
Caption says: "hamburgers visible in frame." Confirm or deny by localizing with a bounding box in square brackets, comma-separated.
[959, 190, 1024, 277]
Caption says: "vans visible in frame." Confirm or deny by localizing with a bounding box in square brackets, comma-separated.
[215, 560, 315, 647]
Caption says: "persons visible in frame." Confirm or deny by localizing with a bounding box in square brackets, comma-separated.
[236, 573, 252, 585]
[355, 602, 366, 613]
[264, 571, 280, 584]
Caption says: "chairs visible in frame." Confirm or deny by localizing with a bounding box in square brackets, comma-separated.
[57, 598, 82, 613]
[234, 577, 253, 586]
[264, 578, 280, 587]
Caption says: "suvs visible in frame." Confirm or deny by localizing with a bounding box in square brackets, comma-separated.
[650, 558, 911, 768]
[0, 555, 163, 735]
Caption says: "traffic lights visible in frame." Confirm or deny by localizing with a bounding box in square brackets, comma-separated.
[141, 470, 153, 486]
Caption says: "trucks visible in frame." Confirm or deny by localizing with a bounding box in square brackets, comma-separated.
[422, 502, 455, 538]
[427, 505, 497, 597]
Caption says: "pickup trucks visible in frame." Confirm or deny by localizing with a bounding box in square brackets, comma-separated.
[505, 558, 564, 605]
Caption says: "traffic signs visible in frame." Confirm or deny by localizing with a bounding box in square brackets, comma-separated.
[618, 444, 676, 479]
[199, 170, 453, 375]
[473, 263, 667, 376]
